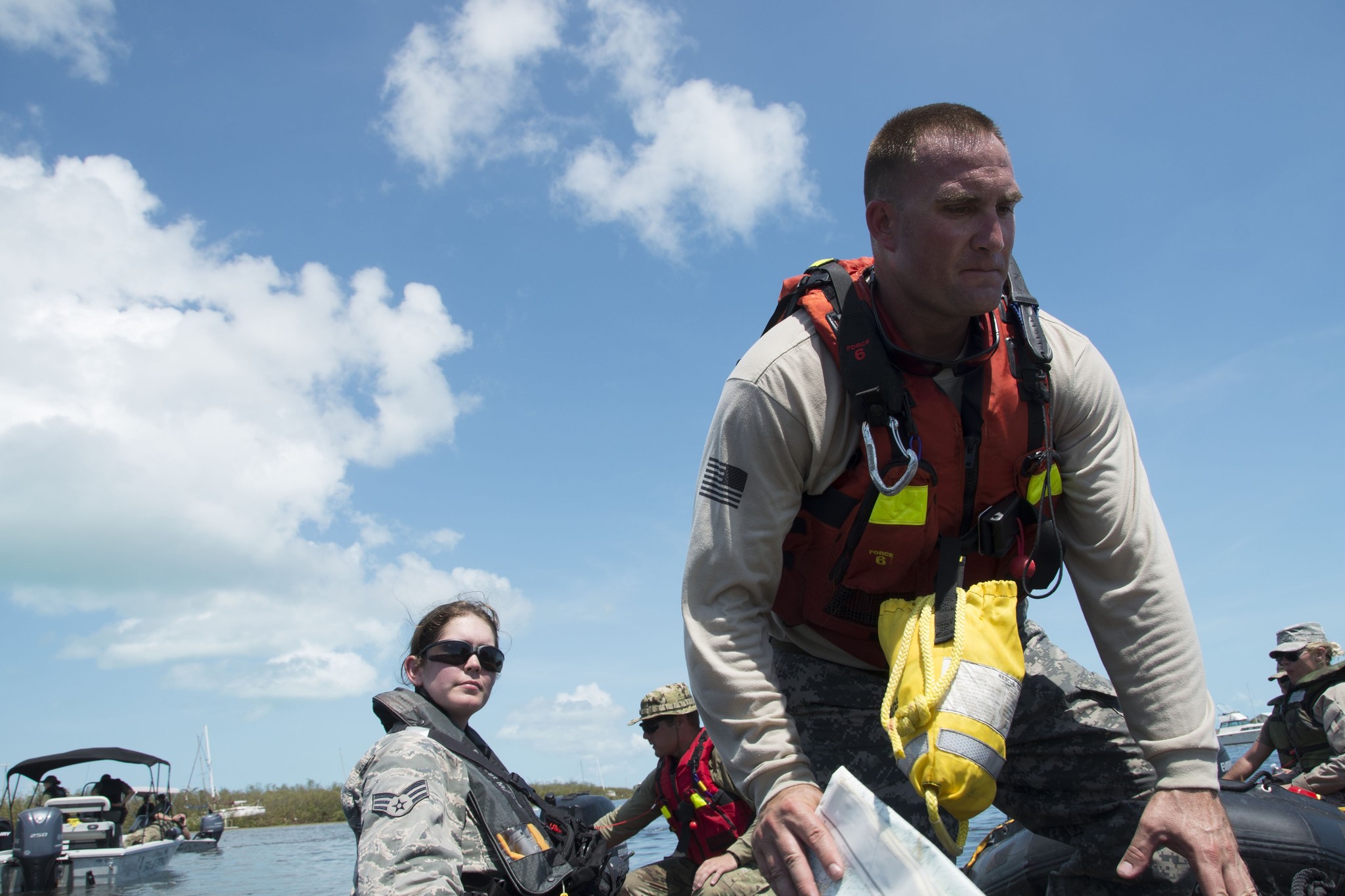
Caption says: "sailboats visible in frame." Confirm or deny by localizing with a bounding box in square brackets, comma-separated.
[184, 723, 267, 819]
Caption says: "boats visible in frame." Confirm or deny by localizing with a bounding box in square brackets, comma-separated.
[1217, 711, 1264, 745]
[0, 746, 187, 896]
[954, 768, 1344, 896]
[175, 808, 226, 852]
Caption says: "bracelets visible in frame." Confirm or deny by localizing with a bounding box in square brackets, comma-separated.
[182, 825, 187, 827]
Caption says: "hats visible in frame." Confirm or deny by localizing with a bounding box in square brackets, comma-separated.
[1269, 621, 1327, 658]
[40, 775, 61, 785]
[627, 681, 697, 726]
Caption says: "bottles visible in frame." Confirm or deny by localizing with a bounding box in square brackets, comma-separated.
[503, 825, 541, 856]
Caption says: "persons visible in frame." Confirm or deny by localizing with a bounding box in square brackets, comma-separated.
[680, 100, 1258, 896]
[339, 599, 573, 896]
[91, 774, 136, 848]
[123, 793, 191, 847]
[588, 681, 777, 896]
[39, 775, 70, 824]
[1219, 622, 1345, 794]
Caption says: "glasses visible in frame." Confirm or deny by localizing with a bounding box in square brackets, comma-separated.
[1276, 648, 1312, 666]
[640, 714, 678, 735]
[417, 639, 505, 673]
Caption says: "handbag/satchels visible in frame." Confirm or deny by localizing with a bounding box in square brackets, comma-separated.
[876, 579, 1025, 856]
